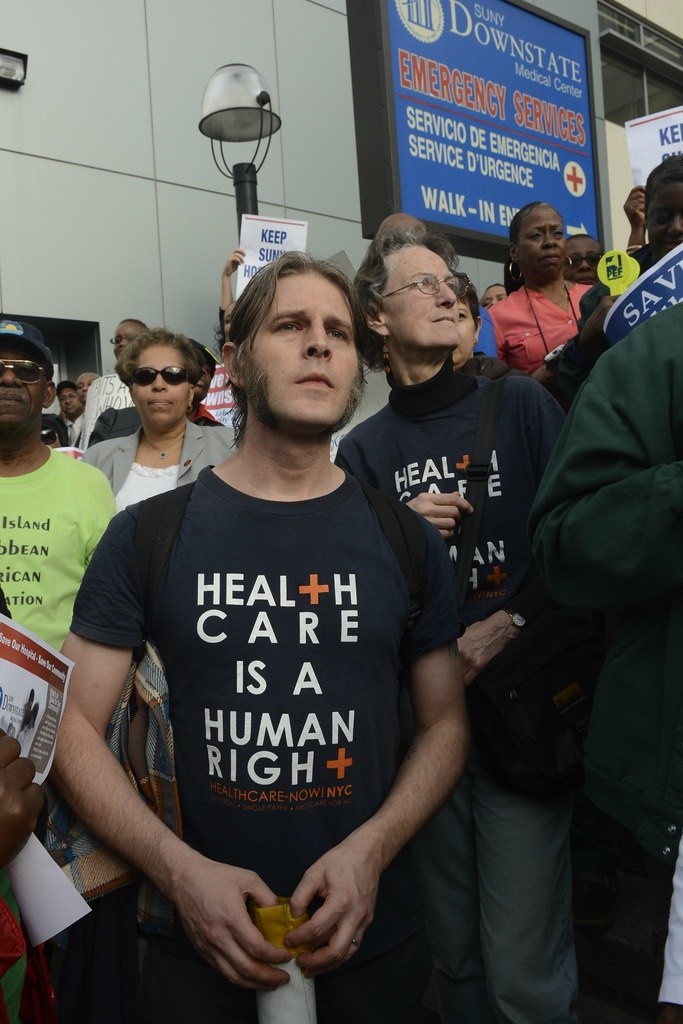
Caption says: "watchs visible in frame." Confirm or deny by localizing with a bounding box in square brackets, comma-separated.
[501, 607, 526, 630]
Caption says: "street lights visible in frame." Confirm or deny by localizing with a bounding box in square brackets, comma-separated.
[196, 62, 283, 250]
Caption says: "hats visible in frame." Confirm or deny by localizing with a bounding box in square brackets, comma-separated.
[41, 414, 69, 447]
[56, 381, 77, 396]
[0, 319, 54, 380]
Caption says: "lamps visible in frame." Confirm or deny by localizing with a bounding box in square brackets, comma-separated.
[0, 46, 29, 90]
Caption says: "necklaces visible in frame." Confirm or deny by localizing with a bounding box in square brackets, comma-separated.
[558, 292, 568, 308]
[144, 436, 184, 458]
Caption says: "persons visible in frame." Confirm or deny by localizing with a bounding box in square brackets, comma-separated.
[0, 156, 682, 1024]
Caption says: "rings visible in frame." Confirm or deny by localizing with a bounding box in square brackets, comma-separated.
[352, 938, 361, 948]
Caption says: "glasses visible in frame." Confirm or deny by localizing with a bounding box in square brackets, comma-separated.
[0, 359, 49, 384]
[41, 429, 58, 445]
[110, 334, 139, 344]
[382, 273, 470, 299]
[564, 253, 602, 269]
[130, 366, 193, 385]
